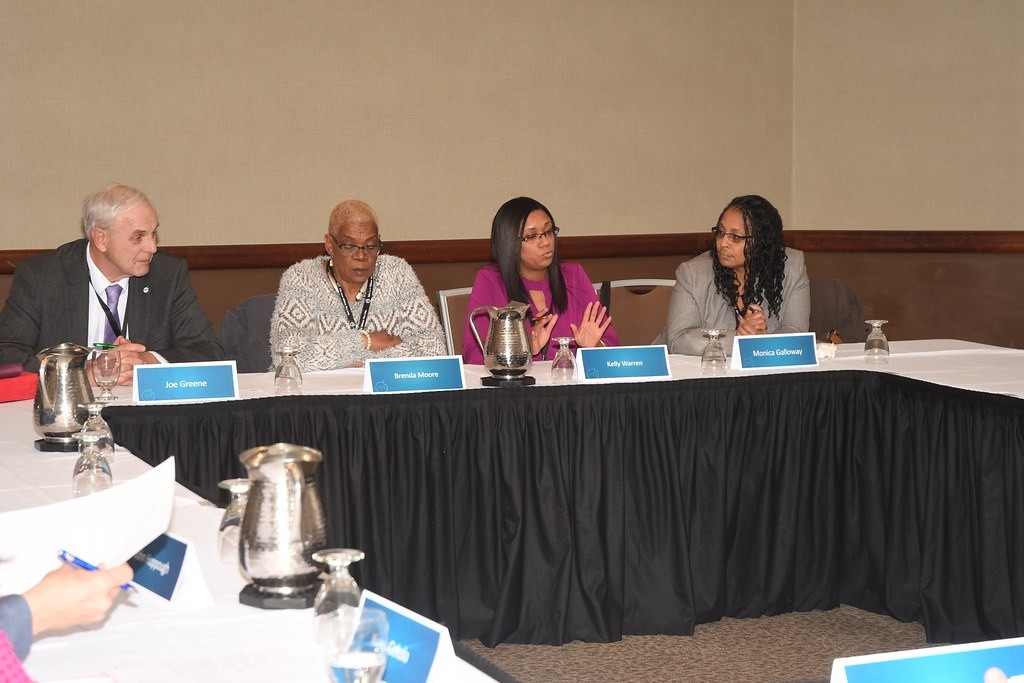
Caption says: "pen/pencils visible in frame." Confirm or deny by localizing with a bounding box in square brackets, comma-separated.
[56, 549, 140, 594]
[531, 317, 543, 320]
[96, 343, 119, 348]
[748, 306, 755, 313]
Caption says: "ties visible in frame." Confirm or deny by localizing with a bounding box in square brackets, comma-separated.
[104, 283, 122, 347]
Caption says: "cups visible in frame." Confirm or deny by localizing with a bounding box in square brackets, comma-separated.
[324, 607, 389, 683]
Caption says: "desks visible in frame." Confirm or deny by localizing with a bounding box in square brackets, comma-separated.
[0, 339, 1023, 683]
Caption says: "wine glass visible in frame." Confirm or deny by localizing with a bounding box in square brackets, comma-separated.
[217, 478, 255, 564]
[71, 432, 112, 496]
[311, 549, 366, 646]
[92, 350, 121, 403]
[274, 350, 303, 395]
[864, 320, 890, 364]
[550, 337, 578, 384]
[700, 329, 727, 375]
[78, 402, 114, 456]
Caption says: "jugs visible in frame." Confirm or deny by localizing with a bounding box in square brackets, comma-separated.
[33, 341, 94, 442]
[468, 300, 533, 379]
[238, 442, 328, 594]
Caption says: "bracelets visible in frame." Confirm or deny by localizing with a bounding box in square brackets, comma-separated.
[360, 330, 371, 350]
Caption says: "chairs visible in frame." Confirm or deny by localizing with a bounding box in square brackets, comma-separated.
[438, 287, 473, 355]
[218, 292, 277, 373]
[595, 280, 677, 346]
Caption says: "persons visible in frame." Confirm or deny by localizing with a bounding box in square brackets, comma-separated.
[0, 184, 226, 387]
[0, 561, 134, 663]
[462, 196, 622, 365]
[269, 199, 448, 372]
[651, 194, 811, 357]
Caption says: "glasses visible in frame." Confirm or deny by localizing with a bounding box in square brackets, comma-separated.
[330, 229, 382, 257]
[711, 224, 752, 243]
[518, 225, 560, 244]
